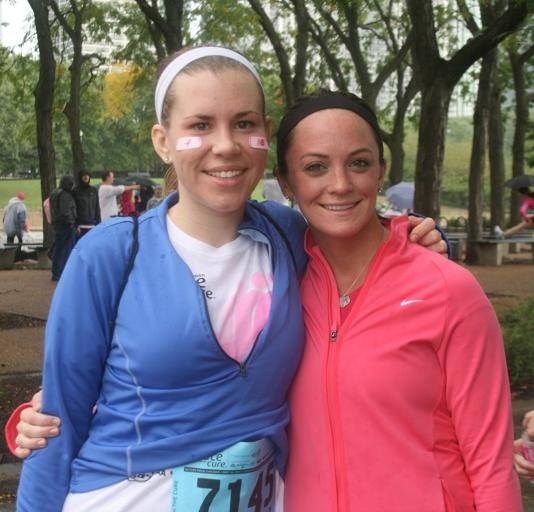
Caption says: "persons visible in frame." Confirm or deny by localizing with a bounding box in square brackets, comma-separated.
[13, 42, 455, 512]
[260, 164, 288, 206]
[2, 168, 168, 283]
[388, 201, 411, 215]
[4, 86, 523, 512]
[491, 187, 534, 240]
[510, 408, 534, 480]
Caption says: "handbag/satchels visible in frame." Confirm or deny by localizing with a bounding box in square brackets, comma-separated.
[43, 197, 53, 224]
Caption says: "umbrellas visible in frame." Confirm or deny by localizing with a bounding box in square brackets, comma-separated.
[504, 173, 534, 192]
[387, 180, 415, 210]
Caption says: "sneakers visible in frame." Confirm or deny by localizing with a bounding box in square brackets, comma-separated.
[494, 225, 504, 240]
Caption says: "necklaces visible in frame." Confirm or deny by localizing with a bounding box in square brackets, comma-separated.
[335, 228, 386, 309]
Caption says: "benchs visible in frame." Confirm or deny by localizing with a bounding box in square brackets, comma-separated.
[444, 229, 533, 266]
